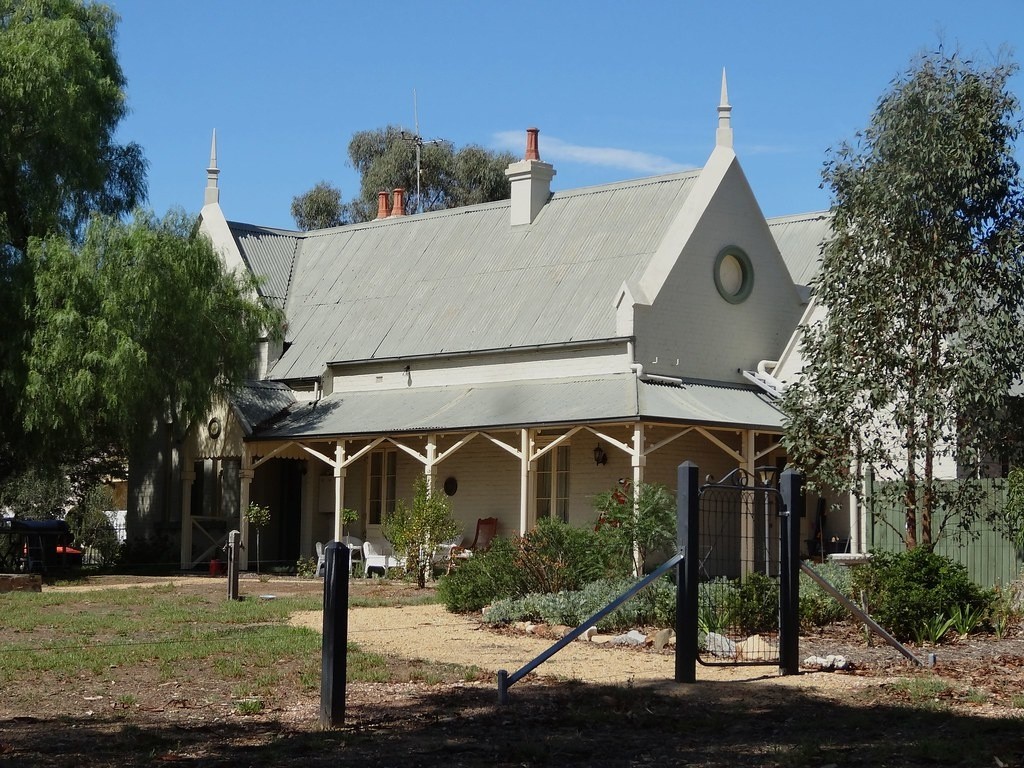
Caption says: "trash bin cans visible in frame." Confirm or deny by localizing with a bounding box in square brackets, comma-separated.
[805, 539, 848, 557]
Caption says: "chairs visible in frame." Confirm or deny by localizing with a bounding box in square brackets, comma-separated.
[316, 517, 498, 579]
[660, 540, 713, 579]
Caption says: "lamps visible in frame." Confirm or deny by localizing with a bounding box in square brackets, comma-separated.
[592, 442, 607, 466]
